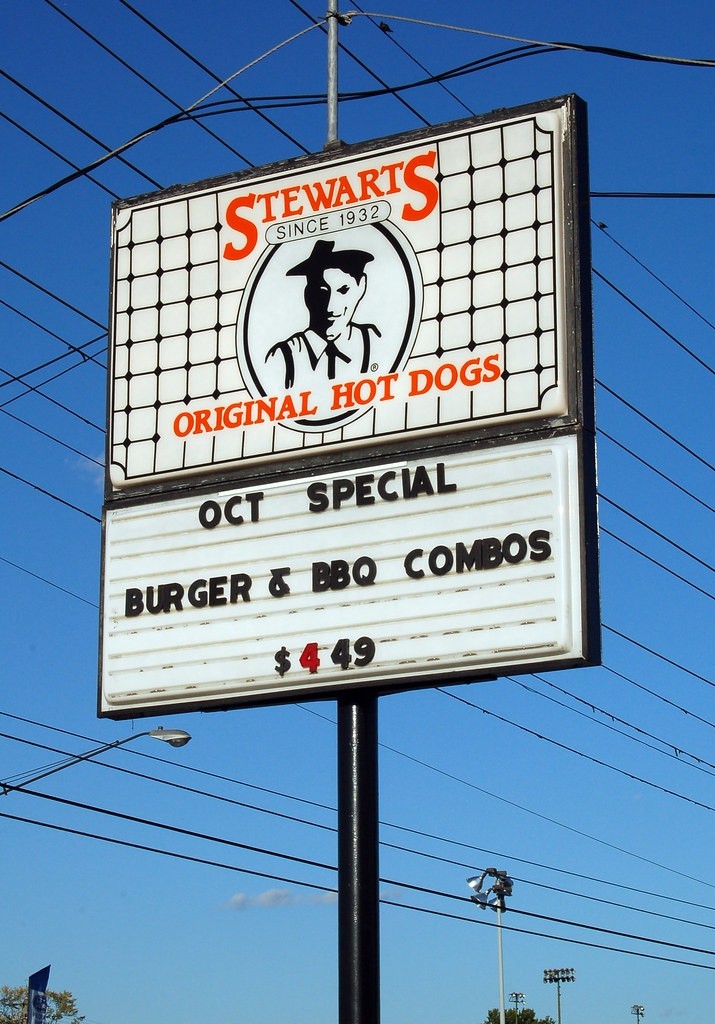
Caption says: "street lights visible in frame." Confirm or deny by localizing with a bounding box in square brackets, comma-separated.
[508, 992, 526, 1024]
[543, 967, 577, 1024]
[467, 866, 509, 1024]
[631, 1005, 646, 1024]
